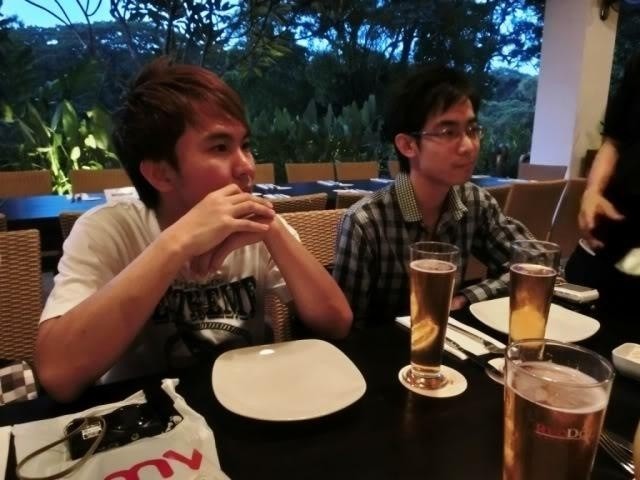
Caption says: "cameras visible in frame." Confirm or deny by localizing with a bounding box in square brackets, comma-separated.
[65, 400, 182, 460]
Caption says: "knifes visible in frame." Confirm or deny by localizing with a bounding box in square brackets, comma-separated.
[446, 338, 503, 378]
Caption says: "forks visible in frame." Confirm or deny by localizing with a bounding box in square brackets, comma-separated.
[449, 321, 506, 353]
[593, 428, 640, 476]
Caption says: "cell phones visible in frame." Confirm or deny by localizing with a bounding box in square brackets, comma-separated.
[554, 282, 600, 304]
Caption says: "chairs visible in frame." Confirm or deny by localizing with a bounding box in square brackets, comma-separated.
[0, 228, 41, 361]
[263, 208, 349, 343]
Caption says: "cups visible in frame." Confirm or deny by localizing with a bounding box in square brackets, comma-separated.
[502, 337, 616, 479]
[500, 239, 565, 379]
[401, 241, 460, 389]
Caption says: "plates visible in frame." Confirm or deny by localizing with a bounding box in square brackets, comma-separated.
[209, 338, 367, 424]
[469, 294, 602, 349]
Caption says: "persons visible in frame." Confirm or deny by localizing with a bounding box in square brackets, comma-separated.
[566, 51, 639, 274]
[332, 67, 550, 321]
[35, 56, 356, 402]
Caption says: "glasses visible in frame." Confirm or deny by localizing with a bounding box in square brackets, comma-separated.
[414, 125, 487, 141]
